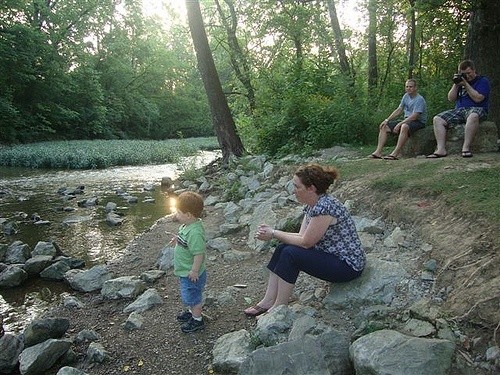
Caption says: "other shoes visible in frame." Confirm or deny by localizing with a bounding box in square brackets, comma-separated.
[177, 309, 205, 332]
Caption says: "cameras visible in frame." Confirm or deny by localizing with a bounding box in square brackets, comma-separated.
[452, 73, 464, 84]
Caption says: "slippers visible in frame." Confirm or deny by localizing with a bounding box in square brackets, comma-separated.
[384, 154, 400, 160]
[461, 151, 473, 158]
[366, 152, 382, 159]
[426, 152, 447, 158]
[244, 302, 268, 316]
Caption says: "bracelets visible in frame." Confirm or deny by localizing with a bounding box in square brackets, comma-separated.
[385, 119, 388, 122]
[272, 230, 274, 237]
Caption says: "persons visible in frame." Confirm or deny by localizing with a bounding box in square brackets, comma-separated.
[245, 163, 367, 320]
[173, 192, 207, 332]
[372, 79, 427, 160]
[426, 60, 491, 158]
